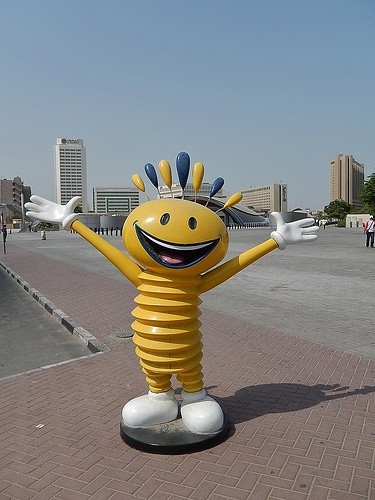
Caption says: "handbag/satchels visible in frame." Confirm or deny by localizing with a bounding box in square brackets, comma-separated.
[366, 230, 369, 234]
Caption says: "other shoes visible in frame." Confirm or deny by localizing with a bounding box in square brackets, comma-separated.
[365, 244, 368, 247]
[369, 245, 373, 248]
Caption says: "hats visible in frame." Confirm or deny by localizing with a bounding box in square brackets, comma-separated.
[369, 215, 373, 218]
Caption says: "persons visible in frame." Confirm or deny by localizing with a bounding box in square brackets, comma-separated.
[3, 224, 32, 242]
[362, 215, 375, 248]
[323, 218, 328, 230]
